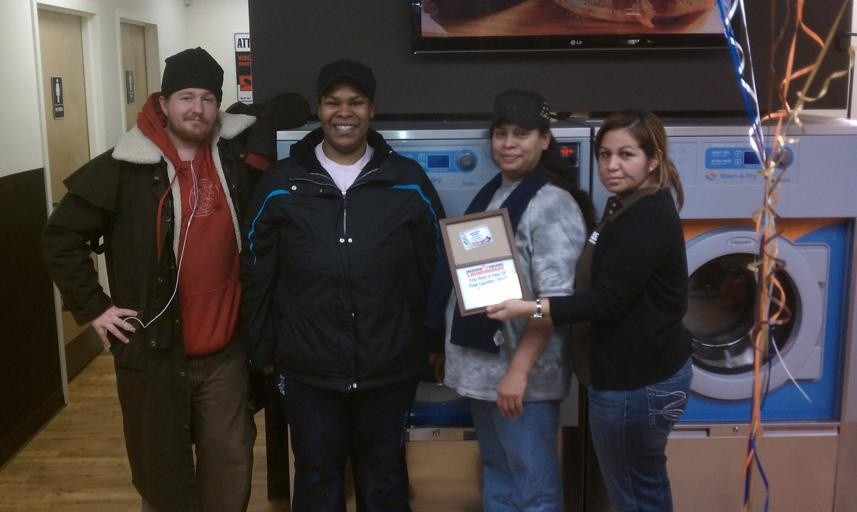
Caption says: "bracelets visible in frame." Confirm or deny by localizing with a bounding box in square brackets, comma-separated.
[531, 295, 545, 320]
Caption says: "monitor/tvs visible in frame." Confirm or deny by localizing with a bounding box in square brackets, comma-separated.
[410, 0, 733, 55]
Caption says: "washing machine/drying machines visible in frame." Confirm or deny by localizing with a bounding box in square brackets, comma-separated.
[276, 119, 591, 428]
[591, 112, 857, 425]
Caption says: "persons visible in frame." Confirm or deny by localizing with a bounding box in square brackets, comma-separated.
[34, 41, 310, 510]
[237, 58, 454, 509]
[483, 108, 695, 509]
[426, 90, 598, 511]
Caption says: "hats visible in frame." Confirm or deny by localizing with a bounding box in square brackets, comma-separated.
[313, 55, 378, 106]
[487, 86, 553, 134]
[159, 45, 227, 107]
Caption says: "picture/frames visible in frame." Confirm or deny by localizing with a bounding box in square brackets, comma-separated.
[438, 206, 533, 316]
[234, 32, 253, 102]
